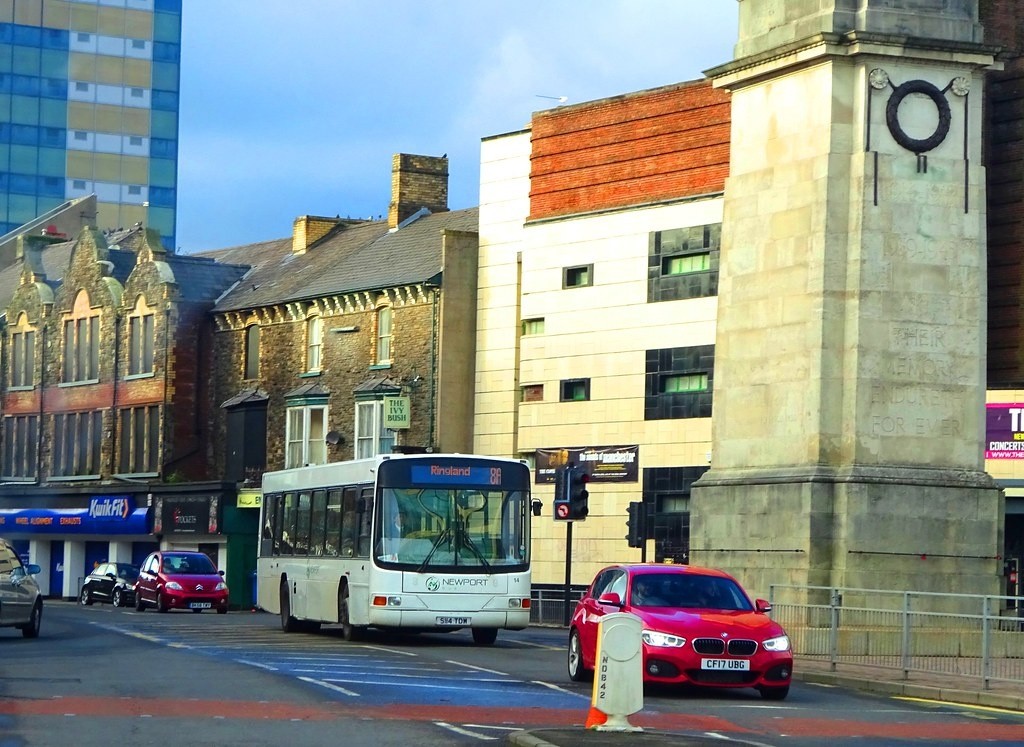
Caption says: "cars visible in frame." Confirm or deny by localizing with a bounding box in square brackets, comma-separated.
[0, 537, 44, 639]
[81, 562, 140, 608]
[134, 550, 229, 613]
[567, 562, 794, 700]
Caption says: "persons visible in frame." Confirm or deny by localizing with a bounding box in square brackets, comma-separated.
[162, 558, 176, 573]
[388, 510, 413, 538]
[690, 580, 723, 607]
[632, 578, 670, 606]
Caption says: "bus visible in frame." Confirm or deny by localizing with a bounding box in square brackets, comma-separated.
[255, 453, 543, 642]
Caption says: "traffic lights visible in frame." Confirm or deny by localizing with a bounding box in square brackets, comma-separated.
[567, 468, 590, 521]
[624, 501, 643, 549]
